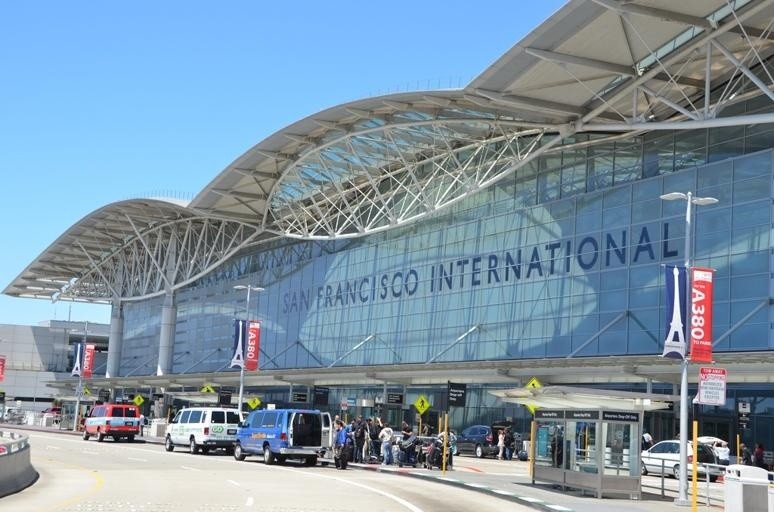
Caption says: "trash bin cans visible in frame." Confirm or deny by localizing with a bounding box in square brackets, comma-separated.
[151, 419, 166, 438]
[42, 413, 53, 427]
[724, 464, 769, 512]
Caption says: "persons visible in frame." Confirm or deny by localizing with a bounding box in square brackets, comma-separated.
[551, 429, 563, 468]
[751, 442, 764, 466]
[740, 443, 751, 465]
[712, 441, 731, 480]
[496, 430, 504, 460]
[140, 412, 145, 436]
[504, 427, 512, 460]
[642, 432, 654, 449]
[438, 427, 457, 471]
[330, 414, 436, 470]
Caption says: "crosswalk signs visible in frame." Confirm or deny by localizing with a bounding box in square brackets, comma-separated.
[413, 395, 429, 414]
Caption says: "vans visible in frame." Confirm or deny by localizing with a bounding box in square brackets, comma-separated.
[164, 407, 244, 456]
[234, 409, 333, 466]
[83, 404, 141, 442]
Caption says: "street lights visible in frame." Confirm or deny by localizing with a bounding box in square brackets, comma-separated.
[658, 190, 719, 506]
[233, 283, 265, 411]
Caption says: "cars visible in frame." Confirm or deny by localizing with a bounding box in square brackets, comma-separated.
[641, 436, 728, 483]
[41, 406, 61, 424]
[393, 434, 438, 451]
[453, 419, 517, 458]
